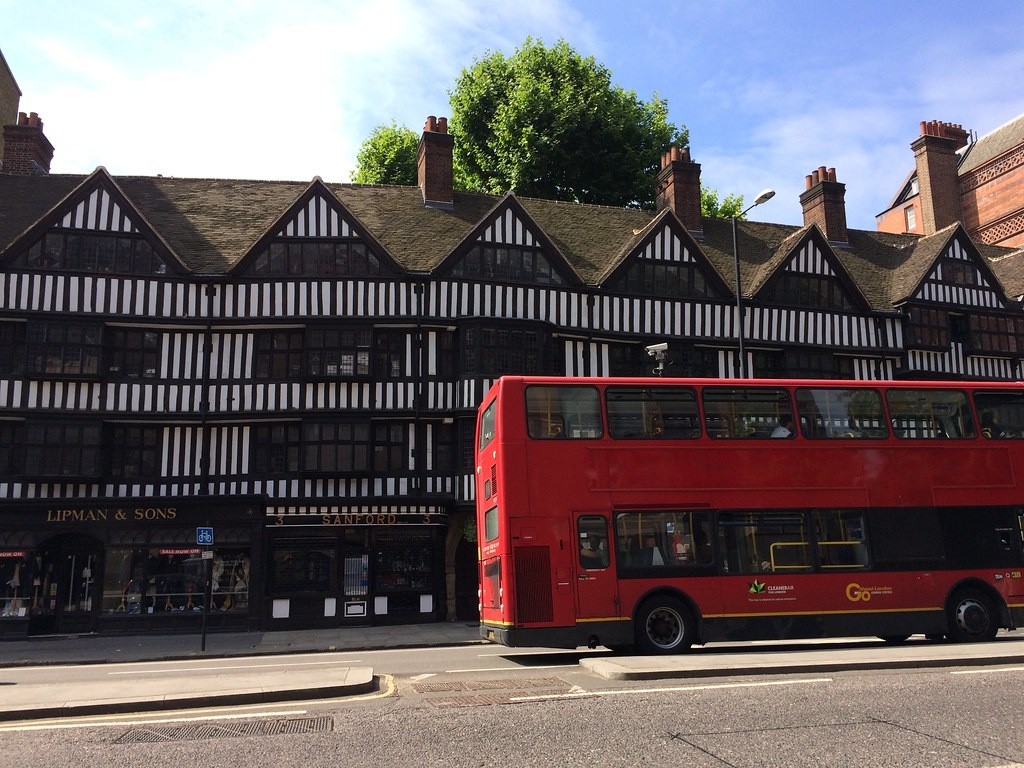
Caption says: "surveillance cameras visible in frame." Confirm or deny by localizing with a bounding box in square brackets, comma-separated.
[646, 343, 668, 353]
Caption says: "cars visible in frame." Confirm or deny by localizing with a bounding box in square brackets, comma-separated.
[121, 572, 232, 614]
[377, 569, 426, 607]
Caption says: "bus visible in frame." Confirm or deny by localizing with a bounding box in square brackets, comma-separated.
[473, 372, 1024, 657]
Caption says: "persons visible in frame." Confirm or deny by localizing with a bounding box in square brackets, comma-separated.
[577, 532, 609, 569]
[982, 410, 1015, 440]
[687, 531, 714, 565]
[770, 413, 796, 440]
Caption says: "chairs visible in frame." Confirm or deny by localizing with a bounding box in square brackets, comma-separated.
[612, 424, 991, 438]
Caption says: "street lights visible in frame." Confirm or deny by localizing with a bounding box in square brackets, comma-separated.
[731, 188, 777, 382]
